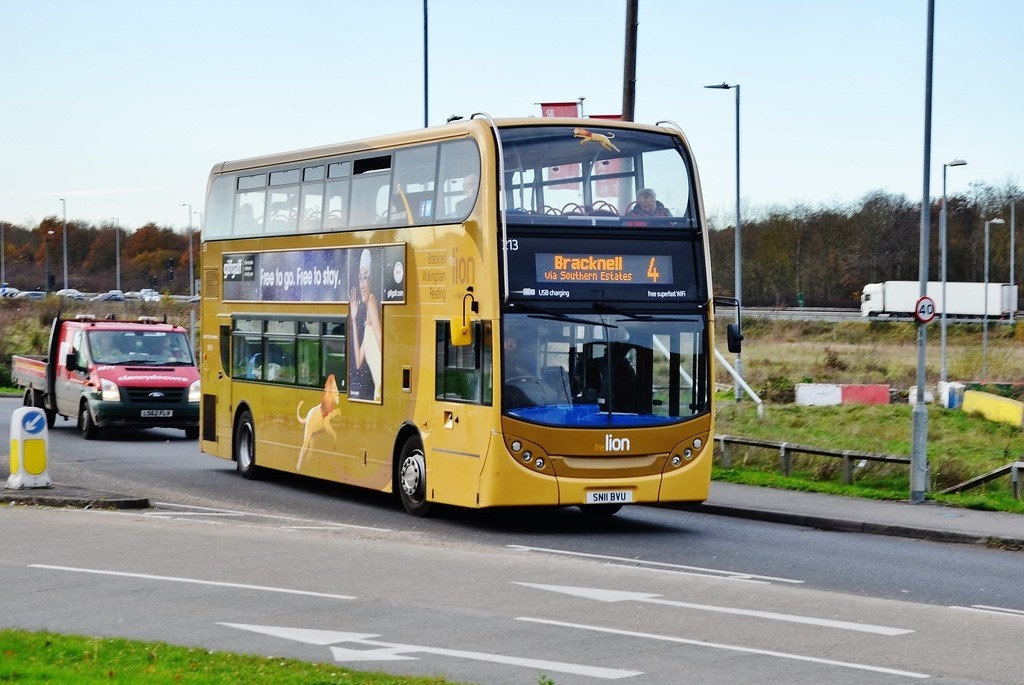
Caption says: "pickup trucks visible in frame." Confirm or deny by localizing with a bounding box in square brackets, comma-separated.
[10, 313, 199, 442]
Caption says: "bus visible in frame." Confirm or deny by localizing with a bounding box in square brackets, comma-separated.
[198, 113, 745, 519]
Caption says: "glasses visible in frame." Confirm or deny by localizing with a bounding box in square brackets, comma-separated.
[358, 273, 370, 283]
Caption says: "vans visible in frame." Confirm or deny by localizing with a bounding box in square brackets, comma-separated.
[14, 292, 47, 300]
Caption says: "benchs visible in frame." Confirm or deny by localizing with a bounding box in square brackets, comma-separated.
[232, 339, 568, 404]
[255, 200, 639, 225]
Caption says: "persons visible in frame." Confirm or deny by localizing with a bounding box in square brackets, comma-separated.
[455, 175, 477, 217]
[626, 188, 672, 225]
[348, 248, 382, 402]
[503, 336, 528, 378]
[150, 336, 181, 359]
[92, 334, 123, 359]
[588, 342, 637, 409]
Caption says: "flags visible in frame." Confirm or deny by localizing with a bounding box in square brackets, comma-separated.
[540, 100, 578, 188]
[590, 113, 623, 197]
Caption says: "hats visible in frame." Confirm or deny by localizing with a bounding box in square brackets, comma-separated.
[359, 248, 372, 271]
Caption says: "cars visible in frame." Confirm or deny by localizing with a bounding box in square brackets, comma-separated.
[55, 289, 83, 300]
[108, 289, 161, 302]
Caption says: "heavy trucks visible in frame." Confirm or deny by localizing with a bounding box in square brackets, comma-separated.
[860, 279, 1018, 321]
